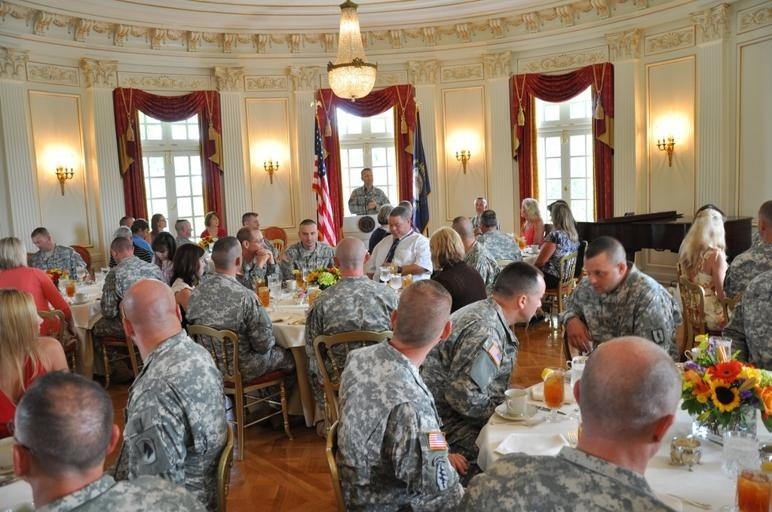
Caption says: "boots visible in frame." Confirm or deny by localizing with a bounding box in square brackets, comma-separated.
[286, 389, 305, 416]
[246, 396, 266, 412]
[112, 358, 134, 384]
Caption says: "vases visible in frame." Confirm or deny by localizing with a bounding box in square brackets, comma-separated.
[707, 408, 757, 446]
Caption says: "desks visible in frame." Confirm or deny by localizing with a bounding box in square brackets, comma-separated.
[54, 280, 106, 380]
[262, 286, 403, 428]
[474, 357, 772, 510]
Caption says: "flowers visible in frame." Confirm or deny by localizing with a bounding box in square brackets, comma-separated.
[45, 268, 69, 289]
[198, 235, 219, 250]
[680, 333, 772, 435]
[306, 265, 342, 291]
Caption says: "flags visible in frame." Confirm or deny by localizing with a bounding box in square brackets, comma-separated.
[313, 114, 337, 249]
[411, 111, 432, 234]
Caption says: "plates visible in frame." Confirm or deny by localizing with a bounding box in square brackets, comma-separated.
[1, 436, 17, 475]
[494, 402, 538, 421]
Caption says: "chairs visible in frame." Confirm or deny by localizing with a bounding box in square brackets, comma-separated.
[325, 419, 347, 512]
[264, 227, 288, 263]
[216, 423, 234, 512]
[101, 301, 144, 390]
[312, 330, 394, 424]
[574, 240, 589, 285]
[675, 262, 745, 363]
[187, 324, 291, 462]
[35, 310, 78, 376]
[523, 251, 579, 334]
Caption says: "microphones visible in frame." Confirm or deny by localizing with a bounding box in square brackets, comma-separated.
[364, 188, 368, 214]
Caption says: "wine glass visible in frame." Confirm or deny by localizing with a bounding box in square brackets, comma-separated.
[379, 263, 414, 295]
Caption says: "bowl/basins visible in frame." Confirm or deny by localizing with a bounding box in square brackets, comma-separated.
[671, 437, 701, 465]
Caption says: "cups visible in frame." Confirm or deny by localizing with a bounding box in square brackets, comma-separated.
[567, 356, 587, 388]
[684, 334, 731, 368]
[514, 235, 540, 256]
[542, 365, 566, 406]
[56, 265, 107, 300]
[505, 389, 528, 416]
[251, 268, 328, 310]
[721, 430, 772, 511]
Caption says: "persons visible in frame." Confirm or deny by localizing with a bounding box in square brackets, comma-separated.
[4, 197, 578, 438]
[348, 167, 391, 215]
[420, 261, 545, 482]
[562, 237, 682, 362]
[112, 277, 229, 510]
[0, 288, 69, 439]
[460, 335, 685, 511]
[676, 200, 772, 370]
[337, 279, 467, 511]
[11, 372, 206, 512]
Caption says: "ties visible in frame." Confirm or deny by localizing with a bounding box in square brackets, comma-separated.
[383, 237, 401, 264]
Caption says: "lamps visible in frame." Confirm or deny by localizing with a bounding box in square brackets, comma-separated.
[56, 167, 74, 196]
[657, 135, 676, 167]
[456, 149, 471, 174]
[264, 160, 278, 184]
[327, 1, 378, 103]
